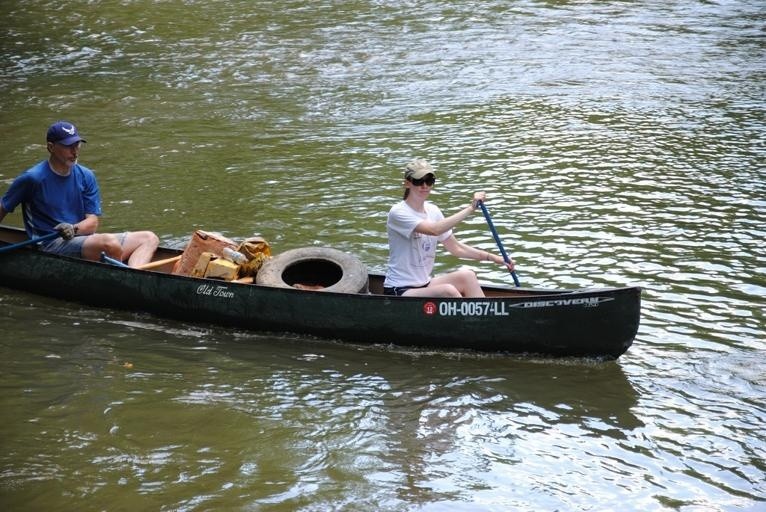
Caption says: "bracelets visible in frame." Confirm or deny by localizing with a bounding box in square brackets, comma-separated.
[72, 222, 78, 237]
[486, 252, 490, 261]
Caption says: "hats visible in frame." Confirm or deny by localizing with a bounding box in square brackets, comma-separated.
[405, 160, 436, 180]
[47, 121, 87, 146]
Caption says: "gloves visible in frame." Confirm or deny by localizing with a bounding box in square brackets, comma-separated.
[53, 222, 76, 241]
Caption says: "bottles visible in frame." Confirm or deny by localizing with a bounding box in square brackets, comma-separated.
[222, 247, 250, 265]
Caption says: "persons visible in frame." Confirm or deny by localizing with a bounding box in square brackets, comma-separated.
[0, 121, 159, 271]
[382, 159, 516, 298]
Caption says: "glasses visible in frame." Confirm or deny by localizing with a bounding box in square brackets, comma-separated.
[412, 178, 435, 186]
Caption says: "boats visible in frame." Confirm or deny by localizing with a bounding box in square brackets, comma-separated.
[0, 226, 642, 361]
